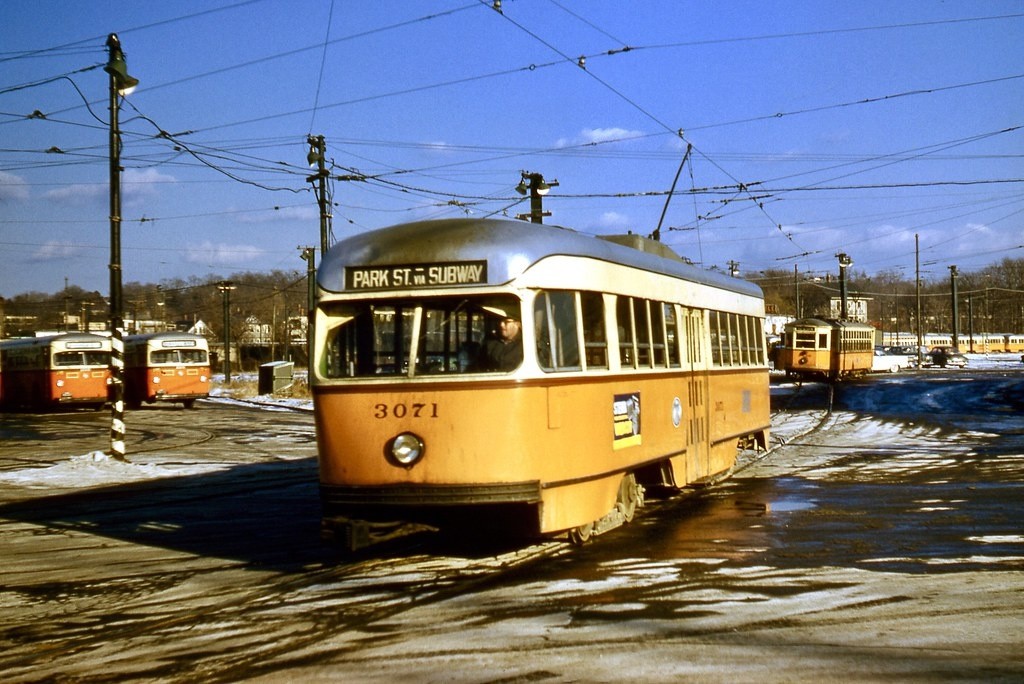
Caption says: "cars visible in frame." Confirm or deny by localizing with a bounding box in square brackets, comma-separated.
[929, 346, 969, 368]
[874, 344, 934, 368]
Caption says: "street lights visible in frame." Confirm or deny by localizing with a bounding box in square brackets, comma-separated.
[964, 293, 976, 354]
[104, 33, 139, 461]
[217, 281, 237, 384]
[126, 300, 145, 336]
[835, 254, 853, 322]
[62, 277, 73, 333]
[297, 245, 316, 391]
[947, 265, 958, 348]
[514, 170, 551, 225]
[305, 135, 330, 256]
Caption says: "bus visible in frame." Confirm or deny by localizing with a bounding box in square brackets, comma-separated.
[0, 333, 112, 411]
[122, 332, 211, 410]
[307, 219, 772, 540]
[783, 318, 876, 382]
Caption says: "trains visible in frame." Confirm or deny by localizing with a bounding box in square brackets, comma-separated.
[883, 337, 1024, 354]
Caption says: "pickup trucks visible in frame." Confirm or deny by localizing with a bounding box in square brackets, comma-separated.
[871, 355, 915, 373]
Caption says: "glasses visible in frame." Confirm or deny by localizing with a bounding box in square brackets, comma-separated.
[496, 317, 516, 324]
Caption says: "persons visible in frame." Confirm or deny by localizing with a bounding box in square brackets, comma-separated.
[468, 304, 548, 373]
